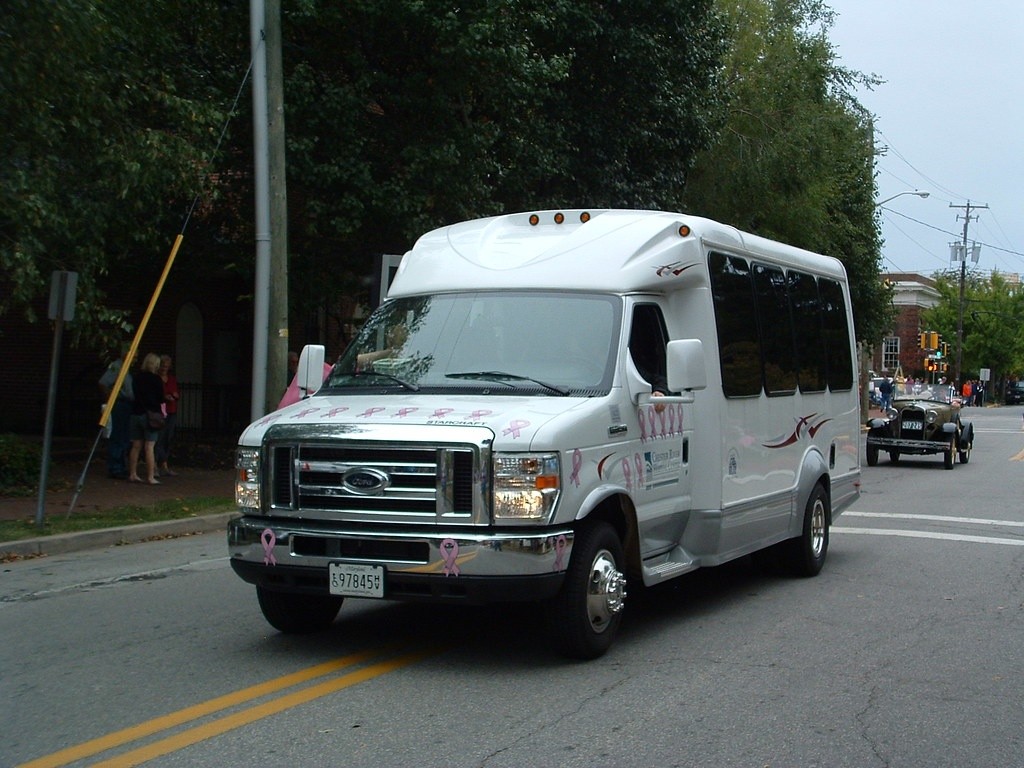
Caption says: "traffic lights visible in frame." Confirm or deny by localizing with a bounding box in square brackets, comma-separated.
[943, 364, 951, 371]
[929, 332, 943, 350]
[917, 333, 926, 350]
[942, 342, 951, 358]
[934, 364, 939, 372]
[924, 358, 934, 373]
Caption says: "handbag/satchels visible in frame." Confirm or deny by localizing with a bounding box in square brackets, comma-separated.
[148, 410, 166, 428]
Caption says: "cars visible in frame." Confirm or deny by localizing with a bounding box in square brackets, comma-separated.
[864, 382, 976, 470]
[1004, 380, 1024, 405]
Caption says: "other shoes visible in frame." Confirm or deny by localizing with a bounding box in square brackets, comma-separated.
[146, 480, 162, 484]
[128, 478, 143, 483]
[154, 471, 159, 477]
[109, 472, 128, 479]
[161, 470, 179, 476]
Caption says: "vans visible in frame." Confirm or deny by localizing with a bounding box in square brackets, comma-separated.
[225, 208, 864, 658]
[870, 376, 907, 405]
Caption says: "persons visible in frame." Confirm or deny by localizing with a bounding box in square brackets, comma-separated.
[593, 324, 673, 412]
[102, 320, 410, 485]
[868, 375, 1016, 434]
